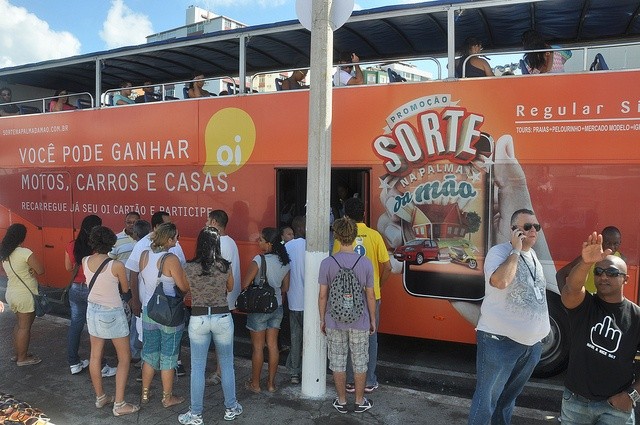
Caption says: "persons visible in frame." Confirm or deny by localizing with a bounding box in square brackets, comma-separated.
[468, 209, 550, 425]
[77, 226, 141, 416]
[128, 219, 152, 243]
[107, 212, 143, 368]
[279, 227, 294, 245]
[280, 67, 310, 90]
[332, 183, 360, 217]
[1, 222, 43, 367]
[523, 31, 573, 74]
[178, 226, 244, 425]
[317, 219, 376, 414]
[48, 89, 77, 112]
[560, 232, 640, 425]
[137, 81, 178, 103]
[284, 217, 307, 384]
[242, 227, 291, 393]
[123, 212, 187, 382]
[206, 210, 241, 388]
[556, 226, 625, 296]
[446, 38, 495, 77]
[332, 52, 364, 85]
[0, 87, 23, 116]
[140, 223, 185, 408]
[112, 81, 137, 106]
[330, 198, 391, 393]
[188, 72, 211, 98]
[65, 215, 118, 379]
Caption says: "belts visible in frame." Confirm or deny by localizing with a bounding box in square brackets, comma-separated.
[192, 306, 229, 316]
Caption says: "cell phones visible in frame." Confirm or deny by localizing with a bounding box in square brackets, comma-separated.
[511, 226, 525, 241]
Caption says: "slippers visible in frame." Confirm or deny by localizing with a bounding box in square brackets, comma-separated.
[17, 355, 42, 367]
[206, 372, 221, 386]
[245, 380, 261, 394]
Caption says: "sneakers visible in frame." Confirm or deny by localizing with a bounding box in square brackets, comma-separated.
[332, 397, 348, 414]
[101, 365, 117, 378]
[224, 403, 243, 421]
[346, 384, 355, 393]
[291, 374, 300, 384]
[365, 381, 378, 392]
[178, 411, 204, 425]
[175, 364, 186, 376]
[354, 397, 374, 413]
[70, 360, 89, 375]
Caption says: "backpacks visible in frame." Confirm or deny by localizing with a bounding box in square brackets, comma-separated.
[326, 255, 365, 324]
[235, 254, 278, 313]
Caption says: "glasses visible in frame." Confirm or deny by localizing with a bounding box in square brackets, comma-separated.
[3, 93, 11, 96]
[594, 267, 626, 277]
[518, 223, 541, 232]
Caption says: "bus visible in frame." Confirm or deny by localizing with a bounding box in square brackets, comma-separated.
[0, 0, 640, 378]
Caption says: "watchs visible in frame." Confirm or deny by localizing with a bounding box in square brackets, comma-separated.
[509, 250, 521, 256]
[625, 386, 640, 403]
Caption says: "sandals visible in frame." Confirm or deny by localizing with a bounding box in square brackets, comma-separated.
[112, 401, 140, 417]
[141, 388, 154, 403]
[161, 392, 185, 408]
[95, 394, 116, 409]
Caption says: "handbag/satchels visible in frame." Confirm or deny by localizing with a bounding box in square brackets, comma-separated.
[60, 263, 79, 306]
[89, 258, 132, 324]
[8, 256, 48, 317]
[147, 253, 190, 327]
